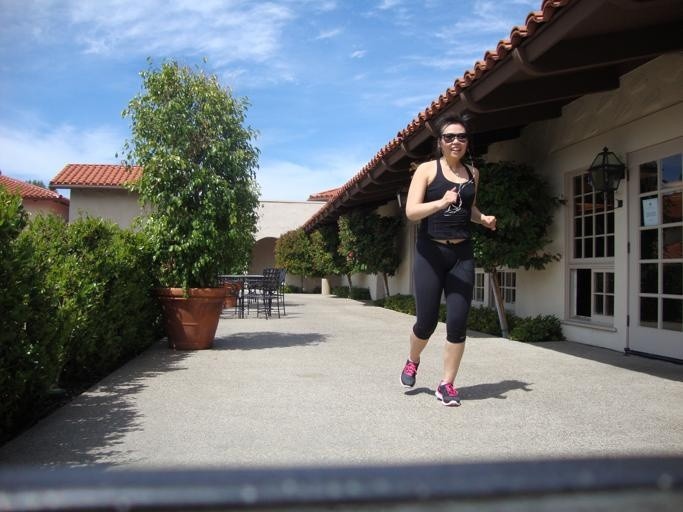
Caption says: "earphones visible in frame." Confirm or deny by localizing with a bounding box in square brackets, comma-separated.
[439, 141, 442, 144]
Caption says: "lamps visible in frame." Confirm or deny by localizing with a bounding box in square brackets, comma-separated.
[587, 146, 626, 193]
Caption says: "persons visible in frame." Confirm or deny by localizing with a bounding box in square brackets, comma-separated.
[400, 111, 497, 405]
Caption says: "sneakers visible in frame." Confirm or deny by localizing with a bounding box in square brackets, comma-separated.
[435, 379, 461, 406]
[400, 353, 420, 387]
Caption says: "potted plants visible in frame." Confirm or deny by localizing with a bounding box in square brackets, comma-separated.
[115, 55, 262, 351]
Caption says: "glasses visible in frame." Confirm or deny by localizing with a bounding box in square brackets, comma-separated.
[442, 133, 468, 142]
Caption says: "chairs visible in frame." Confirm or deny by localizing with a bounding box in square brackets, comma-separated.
[239, 265, 288, 320]
